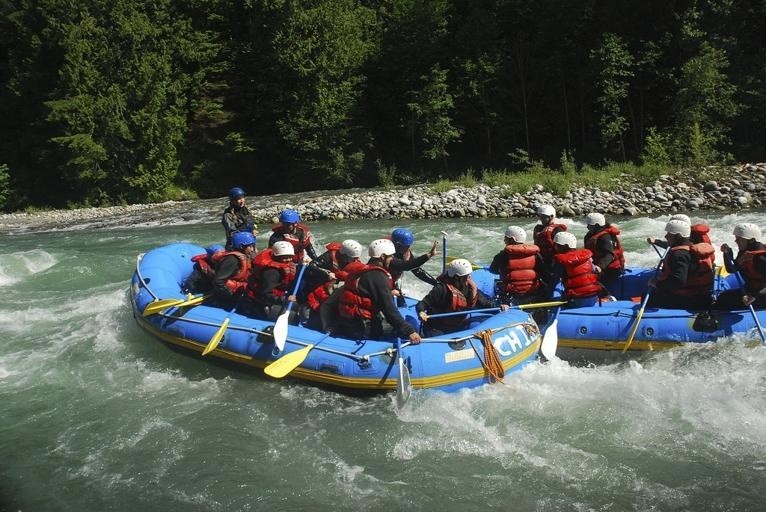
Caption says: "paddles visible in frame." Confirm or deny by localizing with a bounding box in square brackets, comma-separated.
[444, 256, 490, 271]
[393, 294, 411, 410]
[620, 245, 669, 355]
[264, 328, 331, 378]
[272, 257, 308, 351]
[142, 290, 210, 316]
[202, 290, 246, 356]
[424, 301, 569, 320]
[175, 293, 215, 308]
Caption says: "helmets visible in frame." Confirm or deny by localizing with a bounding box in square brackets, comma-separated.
[369, 239, 396, 259]
[233, 232, 256, 249]
[206, 244, 225, 256]
[584, 212, 605, 226]
[733, 223, 761, 243]
[392, 229, 414, 246]
[339, 239, 362, 258]
[270, 240, 295, 257]
[229, 187, 244, 201]
[505, 225, 527, 243]
[553, 232, 577, 249]
[538, 204, 556, 216]
[279, 210, 300, 224]
[665, 219, 691, 238]
[448, 258, 472, 277]
[669, 214, 690, 224]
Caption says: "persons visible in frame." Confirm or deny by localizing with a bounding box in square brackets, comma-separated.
[647, 218, 716, 309]
[714, 222, 766, 311]
[583, 211, 625, 302]
[541, 231, 597, 308]
[648, 213, 715, 264]
[533, 203, 568, 307]
[182, 187, 511, 345]
[488, 226, 547, 309]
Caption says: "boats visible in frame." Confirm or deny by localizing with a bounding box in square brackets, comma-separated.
[440, 253, 765, 351]
[131, 241, 543, 392]
